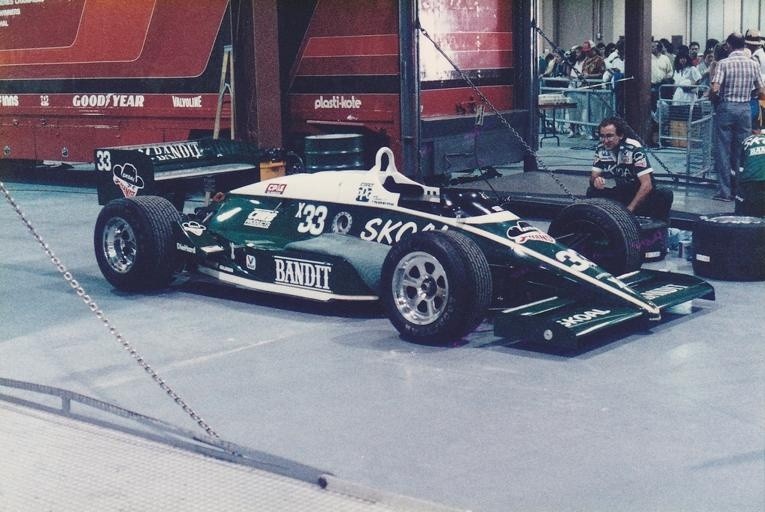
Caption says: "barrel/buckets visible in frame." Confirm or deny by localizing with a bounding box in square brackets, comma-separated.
[304, 135, 363, 171]
[260, 160, 285, 181]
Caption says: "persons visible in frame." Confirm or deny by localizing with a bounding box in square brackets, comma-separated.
[735, 127, 765, 217]
[585, 115, 674, 219]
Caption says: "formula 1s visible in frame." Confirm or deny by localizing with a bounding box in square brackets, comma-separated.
[93, 136, 715, 356]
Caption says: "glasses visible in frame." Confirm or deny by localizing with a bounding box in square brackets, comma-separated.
[599, 136, 619, 142]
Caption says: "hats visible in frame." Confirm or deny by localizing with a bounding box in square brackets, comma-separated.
[581, 40, 596, 52]
[743, 28, 765, 45]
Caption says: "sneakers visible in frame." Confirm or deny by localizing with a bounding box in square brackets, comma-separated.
[711, 192, 733, 202]
[568, 130, 593, 140]
